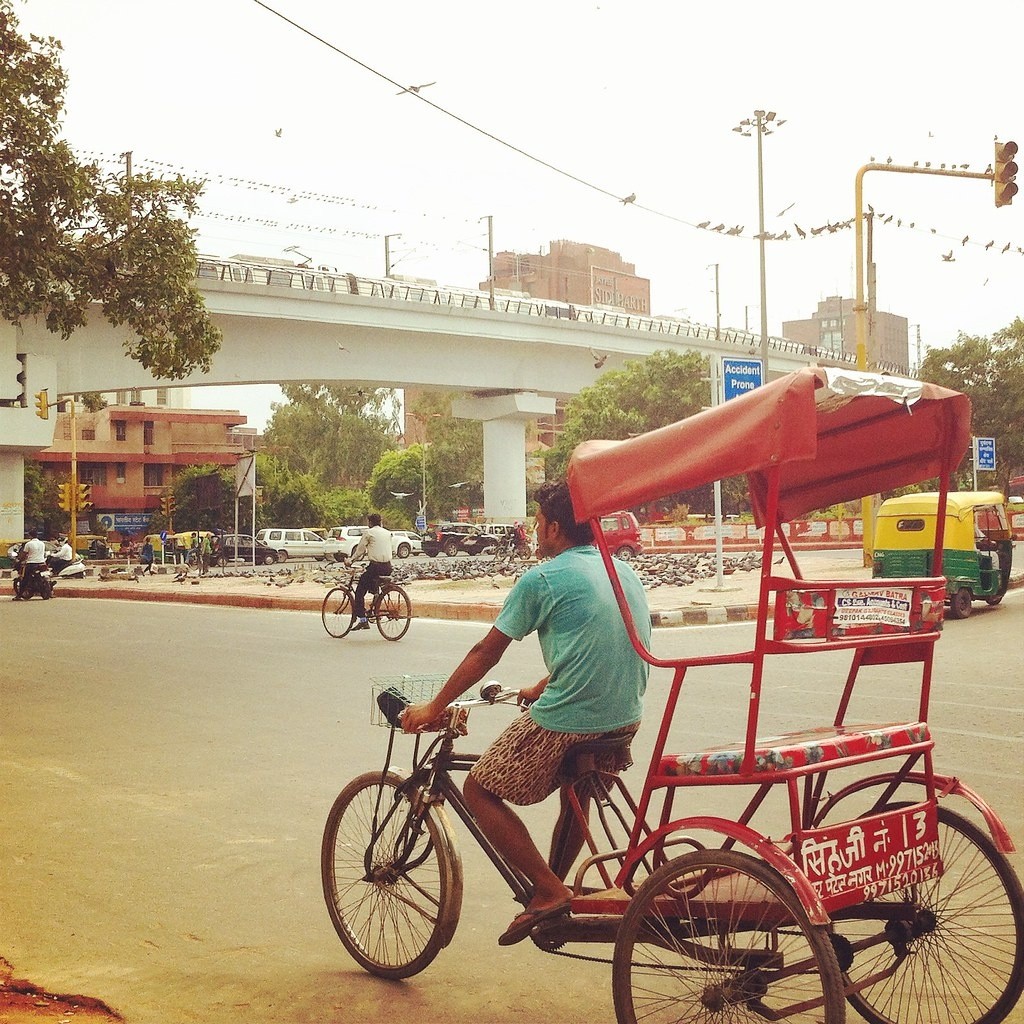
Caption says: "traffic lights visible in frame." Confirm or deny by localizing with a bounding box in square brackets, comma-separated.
[77, 484, 95, 512]
[34, 390, 48, 420]
[160, 497, 167, 518]
[168, 496, 176, 517]
[995, 141, 1019, 208]
[58, 483, 70, 512]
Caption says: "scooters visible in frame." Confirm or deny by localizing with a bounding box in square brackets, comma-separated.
[44, 549, 87, 580]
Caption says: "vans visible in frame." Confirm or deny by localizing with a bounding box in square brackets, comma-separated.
[592, 511, 643, 561]
[256, 529, 335, 563]
[474, 524, 515, 541]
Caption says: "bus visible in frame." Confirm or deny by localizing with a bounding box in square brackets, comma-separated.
[988, 475, 1024, 498]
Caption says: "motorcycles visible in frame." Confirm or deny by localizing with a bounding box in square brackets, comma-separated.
[1006, 497, 1024, 542]
[302, 528, 327, 540]
[494, 534, 531, 560]
[143, 530, 216, 570]
[76, 534, 103, 559]
[873, 491, 1017, 620]
[12, 549, 57, 599]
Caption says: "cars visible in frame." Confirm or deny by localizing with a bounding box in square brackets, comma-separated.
[7, 540, 58, 559]
[390, 529, 425, 557]
[210, 534, 278, 568]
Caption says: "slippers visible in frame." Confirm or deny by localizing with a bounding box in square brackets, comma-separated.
[498, 902, 571, 945]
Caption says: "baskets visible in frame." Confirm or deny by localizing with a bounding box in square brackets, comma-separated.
[367, 675, 474, 736]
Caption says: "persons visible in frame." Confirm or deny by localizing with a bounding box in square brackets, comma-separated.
[185, 533, 198, 564]
[201, 533, 210, 574]
[120, 536, 129, 554]
[508, 521, 526, 561]
[141, 537, 154, 576]
[12, 527, 45, 600]
[401, 483, 650, 946]
[345, 513, 392, 630]
[51, 536, 72, 577]
[973, 510, 1002, 602]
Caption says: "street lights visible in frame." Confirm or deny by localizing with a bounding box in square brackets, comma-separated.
[405, 412, 442, 515]
[731, 109, 788, 384]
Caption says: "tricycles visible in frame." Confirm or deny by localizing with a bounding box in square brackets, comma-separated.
[322, 366, 1023, 1023]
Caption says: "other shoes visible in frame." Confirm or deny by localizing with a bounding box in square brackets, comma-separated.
[351, 621, 370, 630]
[50, 593, 55, 598]
[12, 596, 23, 601]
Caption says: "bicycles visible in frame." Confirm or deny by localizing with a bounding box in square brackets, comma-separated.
[321, 552, 419, 641]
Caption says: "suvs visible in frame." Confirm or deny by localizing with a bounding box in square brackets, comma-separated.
[323, 524, 413, 562]
[420, 522, 501, 557]
